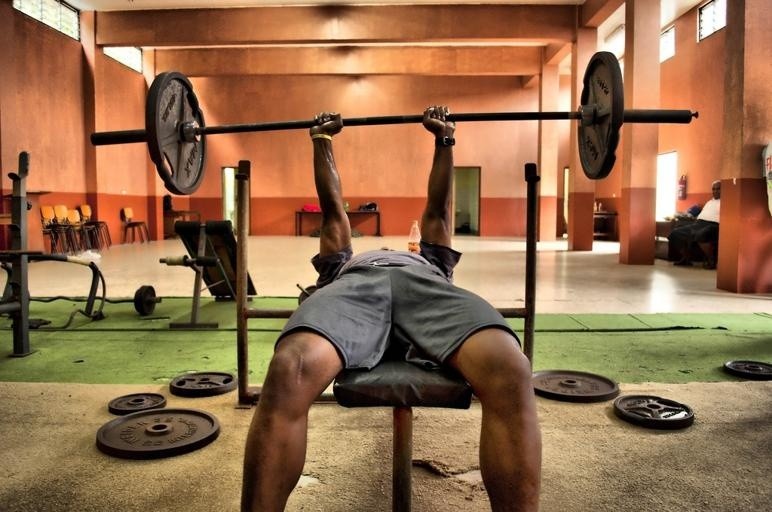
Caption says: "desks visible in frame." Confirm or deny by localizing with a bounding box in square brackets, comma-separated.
[593, 211, 617, 241]
[294, 210, 379, 238]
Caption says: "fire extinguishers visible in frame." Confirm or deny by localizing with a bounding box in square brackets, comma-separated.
[678, 176, 686, 200]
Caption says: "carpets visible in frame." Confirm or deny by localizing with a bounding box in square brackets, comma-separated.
[0, 295, 770, 382]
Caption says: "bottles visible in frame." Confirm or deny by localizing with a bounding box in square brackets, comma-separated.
[593, 201, 602, 212]
[407, 220, 421, 253]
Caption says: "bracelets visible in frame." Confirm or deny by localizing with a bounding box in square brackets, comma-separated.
[434, 136, 455, 146]
[311, 133, 332, 142]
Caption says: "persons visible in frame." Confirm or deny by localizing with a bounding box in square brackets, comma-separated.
[240, 106, 542, 511]
[666, 179, 722, 269]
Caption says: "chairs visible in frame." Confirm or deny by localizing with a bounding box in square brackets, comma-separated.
[39, 204, 153, 256]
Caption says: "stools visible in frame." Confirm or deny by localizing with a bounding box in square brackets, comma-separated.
[331, 363, 473, 510]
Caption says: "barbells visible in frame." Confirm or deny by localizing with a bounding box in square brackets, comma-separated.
[90, 51, 690, 195]
[3, 284, 162, 315]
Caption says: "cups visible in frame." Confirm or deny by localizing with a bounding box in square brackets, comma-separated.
[342, 200, 350, 213]
